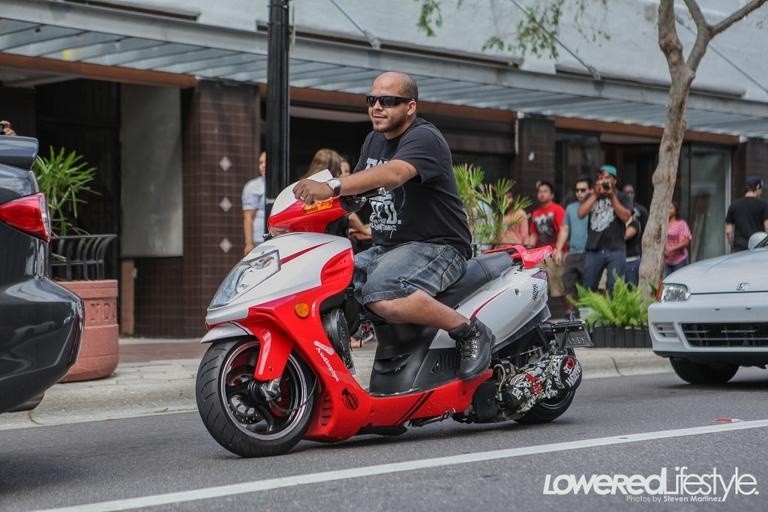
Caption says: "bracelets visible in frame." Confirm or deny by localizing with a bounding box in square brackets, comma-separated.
[325, 178, 341, 198]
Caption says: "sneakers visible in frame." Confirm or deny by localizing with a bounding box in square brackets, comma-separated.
[448, 315, 494, 380]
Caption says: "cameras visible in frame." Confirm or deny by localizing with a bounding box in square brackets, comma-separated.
[602, 181, 609, 191]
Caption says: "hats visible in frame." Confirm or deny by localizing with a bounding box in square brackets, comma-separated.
[743, 176, 763, 191]
[599, 164, 620, 186]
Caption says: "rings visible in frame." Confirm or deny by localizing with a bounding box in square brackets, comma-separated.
[299, 196, 305, 201]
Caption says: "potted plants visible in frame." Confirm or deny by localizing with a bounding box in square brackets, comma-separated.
[27, 142, 123, 385]
[564, 270, 659, 350]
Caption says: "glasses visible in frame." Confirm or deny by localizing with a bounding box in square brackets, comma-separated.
[365, 94, 411, 107]
[576, 188, 586, 192]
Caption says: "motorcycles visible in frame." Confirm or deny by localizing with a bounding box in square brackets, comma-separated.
[192, 170, 593, 456]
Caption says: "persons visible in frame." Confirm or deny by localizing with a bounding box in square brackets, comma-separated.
[240, 151, 266, 259]
[723, 174, 768, 253]
[298, 149, 692, 322]
[0, 120, 17, 137]
[291, 71, 497, 384]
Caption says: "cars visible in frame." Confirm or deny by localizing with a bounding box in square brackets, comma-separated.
[0, 133, 87, 413]
[648, 231, 767, 383]
